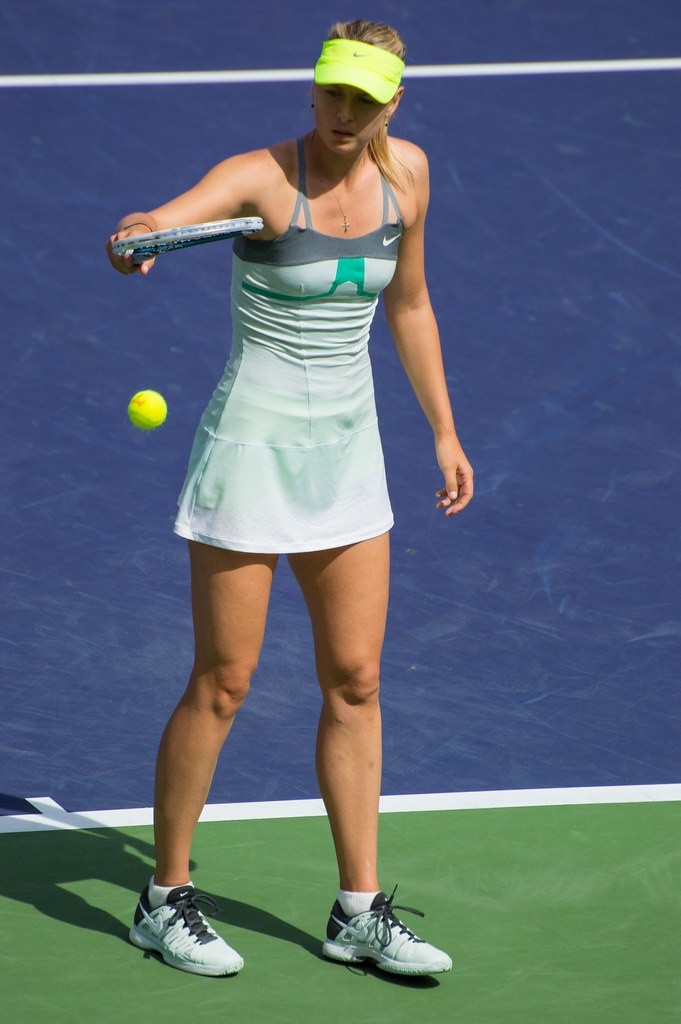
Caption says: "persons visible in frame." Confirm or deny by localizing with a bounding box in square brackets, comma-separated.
[109, 21, 472, 975]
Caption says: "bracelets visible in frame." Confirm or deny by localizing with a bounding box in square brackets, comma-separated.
[121, 223, 153, 236]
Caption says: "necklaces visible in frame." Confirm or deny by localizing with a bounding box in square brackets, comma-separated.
[310, 125, 352, 232]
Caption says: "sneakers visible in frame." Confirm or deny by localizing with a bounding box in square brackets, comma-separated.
[321, 883, 453, 977]
[128, 884, 245, 976]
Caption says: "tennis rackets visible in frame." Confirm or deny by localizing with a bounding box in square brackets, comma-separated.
[111, 216, 266, 268]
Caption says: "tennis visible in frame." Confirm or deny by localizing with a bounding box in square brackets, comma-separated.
[126, 389, 170, 430]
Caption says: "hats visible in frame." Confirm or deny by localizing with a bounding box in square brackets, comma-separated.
[313, 38, 406, 105]
